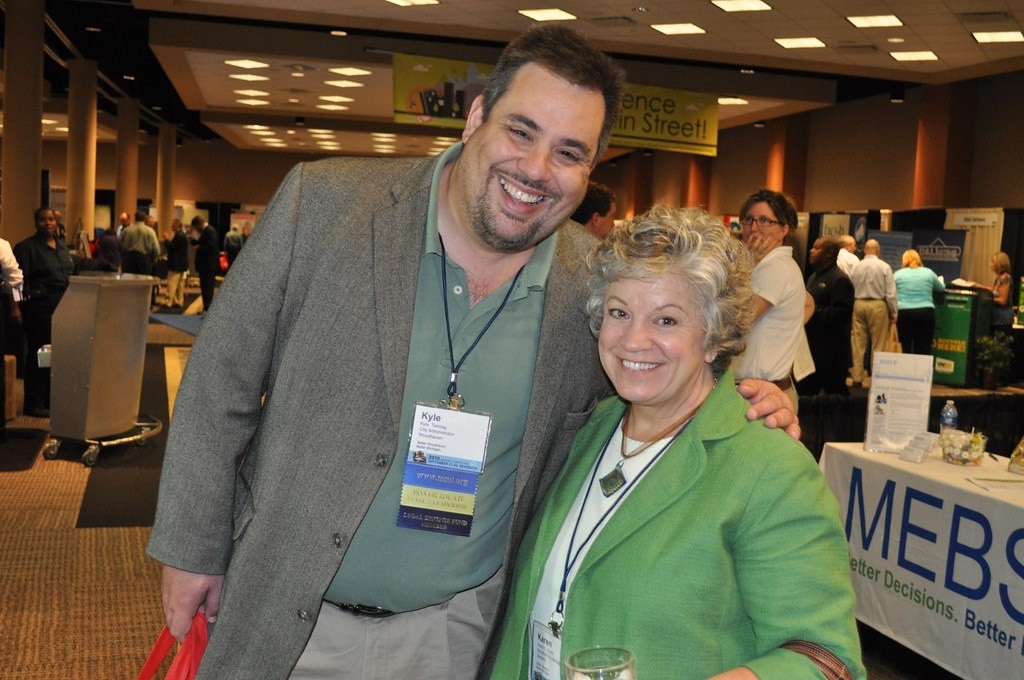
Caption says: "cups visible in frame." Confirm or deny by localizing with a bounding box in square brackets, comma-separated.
[564, 645, 635, 680]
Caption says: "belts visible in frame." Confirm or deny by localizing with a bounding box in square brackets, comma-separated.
[325, 600, 394, 617]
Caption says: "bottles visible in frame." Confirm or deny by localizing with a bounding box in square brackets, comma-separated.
[939, 399, 958, 445]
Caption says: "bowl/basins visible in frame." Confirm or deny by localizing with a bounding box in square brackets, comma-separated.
[941, 431, 989, 466]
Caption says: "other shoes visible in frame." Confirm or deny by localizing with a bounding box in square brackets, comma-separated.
[23, 409, 50, 417]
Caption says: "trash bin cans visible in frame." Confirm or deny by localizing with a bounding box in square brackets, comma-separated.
[39, 273, 163, 467]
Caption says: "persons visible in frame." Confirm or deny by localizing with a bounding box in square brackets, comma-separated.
[736, 188, 945, 416]
[146, 24, 802, 680]
[0, 207, 249, 419]
[570, 181, 618, 238]
[482, 206, 866, 680]
[974, 252, 1014, 386]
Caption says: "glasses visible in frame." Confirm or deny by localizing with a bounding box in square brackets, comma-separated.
[739, 215, 782, 227]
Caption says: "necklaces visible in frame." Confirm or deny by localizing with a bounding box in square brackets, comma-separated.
[600, 407, 706, 498]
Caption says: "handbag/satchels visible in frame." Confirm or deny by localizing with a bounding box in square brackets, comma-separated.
[886, 324, 901, 352]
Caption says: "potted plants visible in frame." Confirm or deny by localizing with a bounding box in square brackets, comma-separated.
[973, 332, 1012, 389]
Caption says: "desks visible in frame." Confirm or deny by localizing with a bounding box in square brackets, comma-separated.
[815, 435, 1024, 680]
[833, 378, 1023, 452]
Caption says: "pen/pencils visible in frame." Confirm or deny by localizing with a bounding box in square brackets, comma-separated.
[989, 453, 998, 461]
